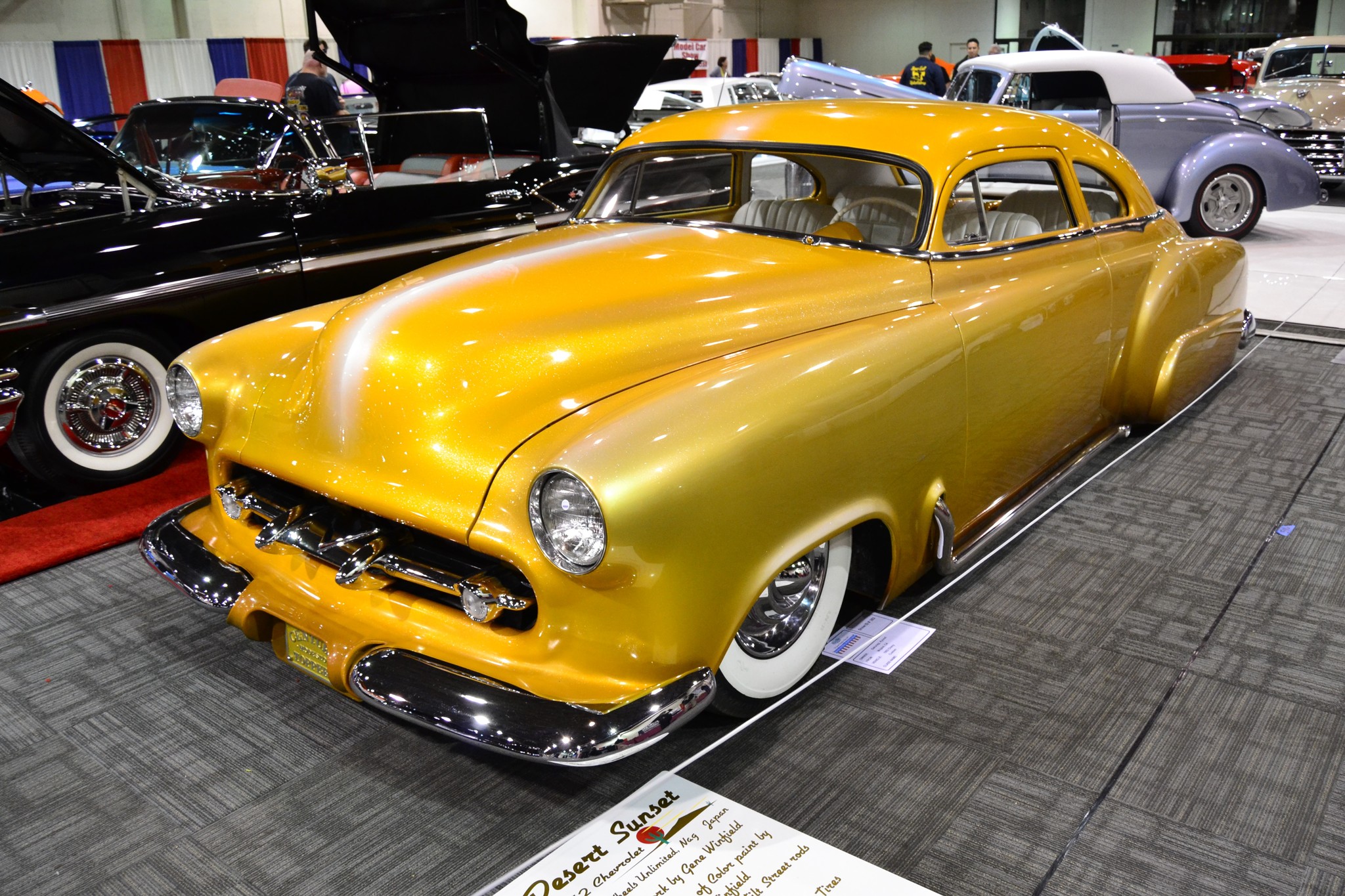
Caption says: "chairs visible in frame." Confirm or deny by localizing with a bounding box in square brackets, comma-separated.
[732, 199, 838, 233]
[943, 210, 1042, 245]
[365, 171, 442, 187]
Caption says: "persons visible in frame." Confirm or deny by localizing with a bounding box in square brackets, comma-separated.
[899, 42, 950, 98]
[284, 39, 359, 156]
[951, 38, 981, 85]
[710, 57, 730, 77]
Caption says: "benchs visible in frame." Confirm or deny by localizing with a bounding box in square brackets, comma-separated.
[832, 184, 1117, 247]
[401, 155, 539, 177]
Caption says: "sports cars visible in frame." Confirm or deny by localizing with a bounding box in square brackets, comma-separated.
[136, 95, 1261, 774]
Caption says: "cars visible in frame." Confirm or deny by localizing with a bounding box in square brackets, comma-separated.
[0, 74, 736, 504]
[106, 1, 1345, 241]
